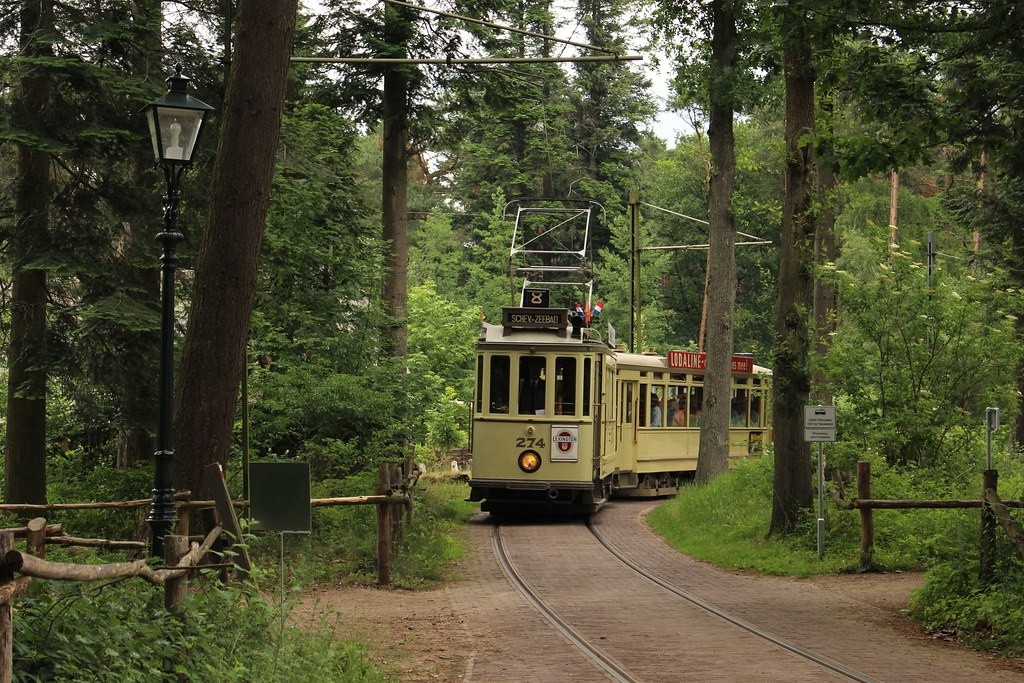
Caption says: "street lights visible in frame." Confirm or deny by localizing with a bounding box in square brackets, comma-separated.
[137, 60, 217, 558]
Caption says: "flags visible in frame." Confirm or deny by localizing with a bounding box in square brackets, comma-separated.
[591, 299, 603, 314]
[576, 302, 585, 319]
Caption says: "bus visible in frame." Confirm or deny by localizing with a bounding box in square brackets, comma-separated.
[463, 288, 774, 519]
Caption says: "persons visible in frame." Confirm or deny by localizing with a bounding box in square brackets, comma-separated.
[731, 395, 760, 427]
[519, 365, 546, 415]
[678, 393, 702, 427]
[651, 393, 662, 427]
[668, 399, 684, 427]
